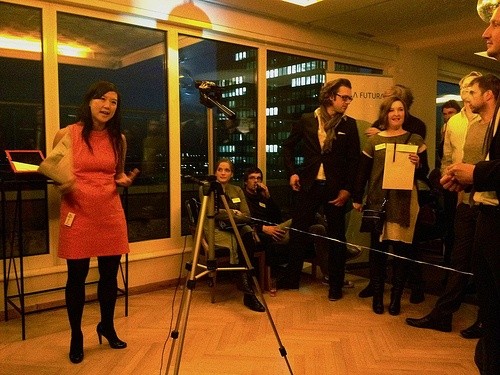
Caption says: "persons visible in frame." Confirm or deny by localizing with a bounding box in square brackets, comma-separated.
[358, 84, 427, 303]
[53, 82, 139, 364]
[199, 159, 265, 312]
[405, 2, 500, 375]
[242, 166, 292, 277]
[314, 212, 365, 288]
[352, 97, 430, 316]
[280, 79, 360, 300]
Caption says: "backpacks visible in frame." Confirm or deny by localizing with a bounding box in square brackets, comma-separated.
[215, 192, 246, 230]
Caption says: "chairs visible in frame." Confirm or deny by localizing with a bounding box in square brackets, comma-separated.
[187, 198, 318, 292]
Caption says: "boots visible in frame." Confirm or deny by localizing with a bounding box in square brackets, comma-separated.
[389, 276, 407, 315]
[371, 274, 386, 314]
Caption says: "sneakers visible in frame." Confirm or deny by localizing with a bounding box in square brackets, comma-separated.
[322, 274, 354, 288]
[345, 246, 363, 262]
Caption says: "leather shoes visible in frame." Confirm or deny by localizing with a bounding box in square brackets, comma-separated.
[236, 271, 254, 295]
[460, 322, 482, 339]
[406, 314, 453, 333]
[244, 294, 266, 312]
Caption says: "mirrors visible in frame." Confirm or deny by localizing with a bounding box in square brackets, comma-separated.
[5, 150, 45, 173]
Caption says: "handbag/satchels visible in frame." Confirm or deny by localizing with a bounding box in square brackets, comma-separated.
[359, 201, 388, 235]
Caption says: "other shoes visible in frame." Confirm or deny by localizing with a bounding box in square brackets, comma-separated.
[271, 276, 299, 290]
[328, 286, 343, 301]
[409, 290, 424, 303]
[359, 282, 373, 298]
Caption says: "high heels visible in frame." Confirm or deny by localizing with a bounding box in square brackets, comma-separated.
[96, 321, 128, 349]
[69, 331, 85, 364]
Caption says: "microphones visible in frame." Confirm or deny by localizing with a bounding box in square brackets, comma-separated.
[183, 175, 212, 190]
[195, 81, 235, 120]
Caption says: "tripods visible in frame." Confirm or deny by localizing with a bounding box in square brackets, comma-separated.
[164, 182, 294, 375]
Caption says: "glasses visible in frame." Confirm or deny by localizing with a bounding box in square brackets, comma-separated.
[336, 92, 353, 103]
[249, 177, 262, 182]
